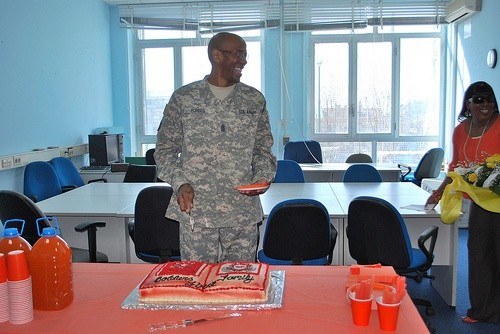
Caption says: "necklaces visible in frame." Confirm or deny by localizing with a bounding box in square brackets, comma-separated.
[463, 119, 490, 164]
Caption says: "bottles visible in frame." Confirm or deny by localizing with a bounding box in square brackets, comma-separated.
[0, 219, 33, 256]
[30, 216, 74, 311]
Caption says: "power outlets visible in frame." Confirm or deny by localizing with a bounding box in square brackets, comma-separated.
[283, 136, 289, 145]
[14, 156, 23, 166]
[68, 148, 75, 156]
[60, 149, 68, 157]
[2, 158, 13, 169]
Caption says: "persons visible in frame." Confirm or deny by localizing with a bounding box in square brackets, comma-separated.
[425, 81, 500, 323]
[153, 32, 277, 262]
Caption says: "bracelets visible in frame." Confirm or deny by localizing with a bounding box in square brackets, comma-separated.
[432, 190, 442, 200]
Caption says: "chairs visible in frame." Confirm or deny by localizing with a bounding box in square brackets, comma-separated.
[0, 137, 445, 315]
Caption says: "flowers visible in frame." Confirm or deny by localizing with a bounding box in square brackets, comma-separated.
[448, 153, 500, 197]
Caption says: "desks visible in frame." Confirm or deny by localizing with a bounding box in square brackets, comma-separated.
[421, 171, 471, 228]
[117, 183, 345, 267]
[300, 162, 401, 182]
[36, 182, 155, 264]
[329, 182, 464, 306]
[0, 263, 434, 334]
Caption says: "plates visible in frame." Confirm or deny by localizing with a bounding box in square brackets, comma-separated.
[237, 184, 271, 194]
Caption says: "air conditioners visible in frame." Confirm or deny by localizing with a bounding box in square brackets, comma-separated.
[444, 0, 482, 24]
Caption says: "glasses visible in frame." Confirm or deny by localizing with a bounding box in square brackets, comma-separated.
[468, 95, 495, 104]
[217, 48, 247, 59]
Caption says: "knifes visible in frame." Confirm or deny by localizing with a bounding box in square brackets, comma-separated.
[148, 312, 242, 333]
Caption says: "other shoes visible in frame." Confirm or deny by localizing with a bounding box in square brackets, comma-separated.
[462, 316, 478, 322]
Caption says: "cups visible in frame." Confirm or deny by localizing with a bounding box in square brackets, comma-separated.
[349, 291, 374, 327]
[376, 296, 402, 332]
[0, 249, 34, 325]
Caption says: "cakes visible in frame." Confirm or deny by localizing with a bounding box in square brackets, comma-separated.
[138, 260, 271, 303]
